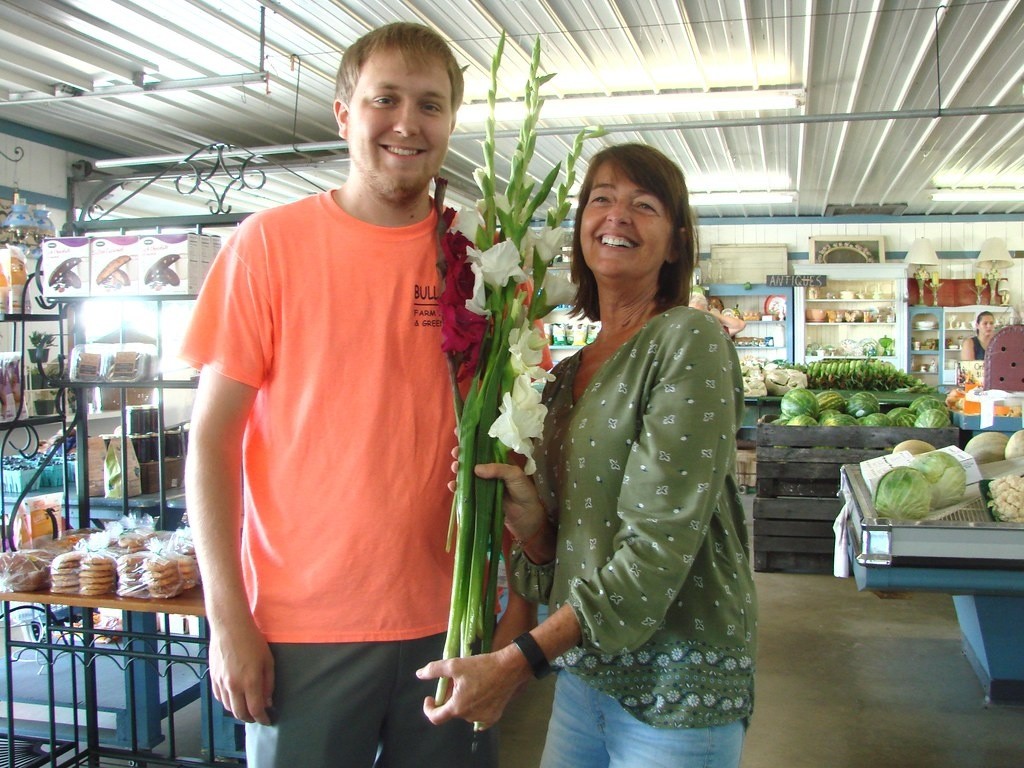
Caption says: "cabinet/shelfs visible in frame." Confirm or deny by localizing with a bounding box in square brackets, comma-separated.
[942, 305, 1014, 385]
[701, 284, 793, 367]
[792, 264, 907, 375]
[541, 262, 602, 348]
[907, 306, 943, 393]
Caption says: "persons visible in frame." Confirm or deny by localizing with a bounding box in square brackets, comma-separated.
[961, 311, 995, 360]
[416, 144, 758, 768]
[177, 21, 552, 768]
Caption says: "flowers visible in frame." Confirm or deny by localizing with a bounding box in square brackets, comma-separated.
[433, 28, 610, 729]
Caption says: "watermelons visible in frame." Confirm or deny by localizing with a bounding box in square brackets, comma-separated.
[769, 388, 951, 450]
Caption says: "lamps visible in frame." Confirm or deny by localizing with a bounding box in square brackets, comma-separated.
[975, 236, 1015, 305]
[28, 202, 56, 244]
[0, 198, 38, 240]
[905, 236, 940, 306]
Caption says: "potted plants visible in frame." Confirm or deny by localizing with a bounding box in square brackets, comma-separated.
[29, 331, 56, 362]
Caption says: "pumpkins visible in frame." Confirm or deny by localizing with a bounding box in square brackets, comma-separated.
[893, 429, 1024, 464]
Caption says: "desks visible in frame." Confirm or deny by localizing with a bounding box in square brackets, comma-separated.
[0, 580, 207, 768]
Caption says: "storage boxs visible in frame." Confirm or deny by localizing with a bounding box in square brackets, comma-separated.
[138, 232, 223, 296]
[752, 415, 993, 576]
[90, 236, 140, 295]
[43, 236, 89, 296]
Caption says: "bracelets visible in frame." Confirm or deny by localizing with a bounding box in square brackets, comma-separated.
[515, 633, 552, 678]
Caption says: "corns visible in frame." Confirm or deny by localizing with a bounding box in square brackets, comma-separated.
[780, 357, 938, 393]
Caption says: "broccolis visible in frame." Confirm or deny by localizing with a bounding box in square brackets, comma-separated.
[987, 474, 1024, 524]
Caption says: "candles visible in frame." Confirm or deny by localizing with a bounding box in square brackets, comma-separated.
[931, 271, 939, 286]
[975, 272, 982, 286]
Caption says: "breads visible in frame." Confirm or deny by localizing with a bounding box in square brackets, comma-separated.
[48, 253, 181, 288]
[0, 529, 198, 600]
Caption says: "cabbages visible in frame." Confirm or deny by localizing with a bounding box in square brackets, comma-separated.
[875, 451, 966, 521]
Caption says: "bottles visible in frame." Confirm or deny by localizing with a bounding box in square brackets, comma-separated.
[733, 304, 741, 319]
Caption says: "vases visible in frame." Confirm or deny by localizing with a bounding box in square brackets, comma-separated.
[34, 399, 55, 414]
